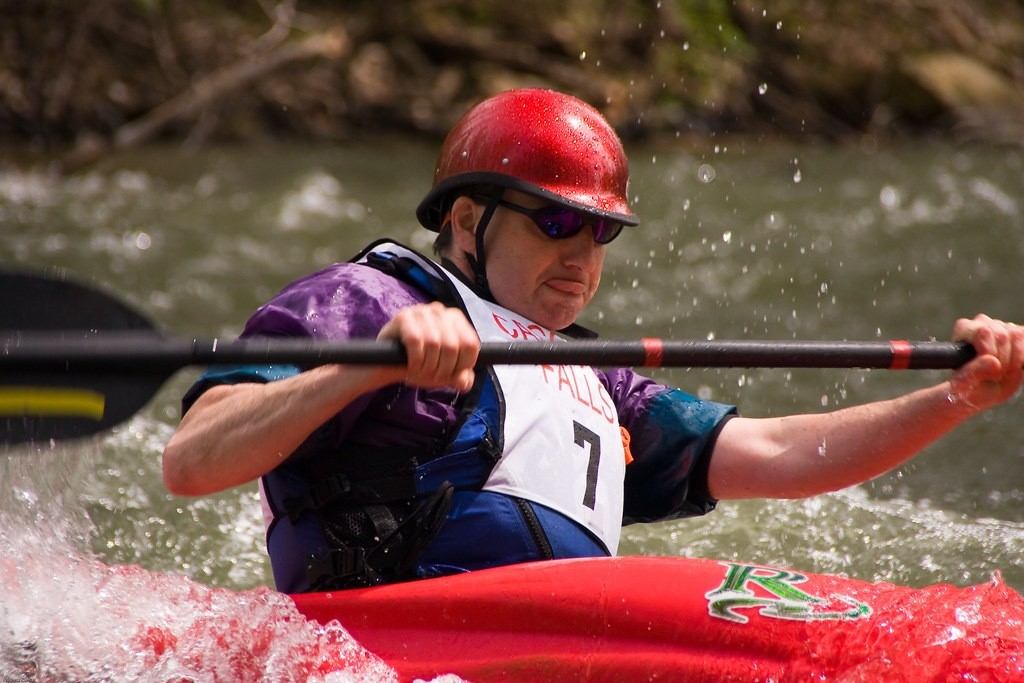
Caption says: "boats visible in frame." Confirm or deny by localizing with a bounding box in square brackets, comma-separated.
[1, 546, 1024, 683]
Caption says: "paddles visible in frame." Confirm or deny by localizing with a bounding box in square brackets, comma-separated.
[0, 263, 972, 443]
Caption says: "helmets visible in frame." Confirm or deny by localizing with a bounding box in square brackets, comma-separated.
[415, 87, 641, 233]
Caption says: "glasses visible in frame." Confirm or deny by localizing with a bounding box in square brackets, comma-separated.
[468, 190, 624, 244]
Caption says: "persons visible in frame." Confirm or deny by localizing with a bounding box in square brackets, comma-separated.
[163, 88, 1024, 592]
